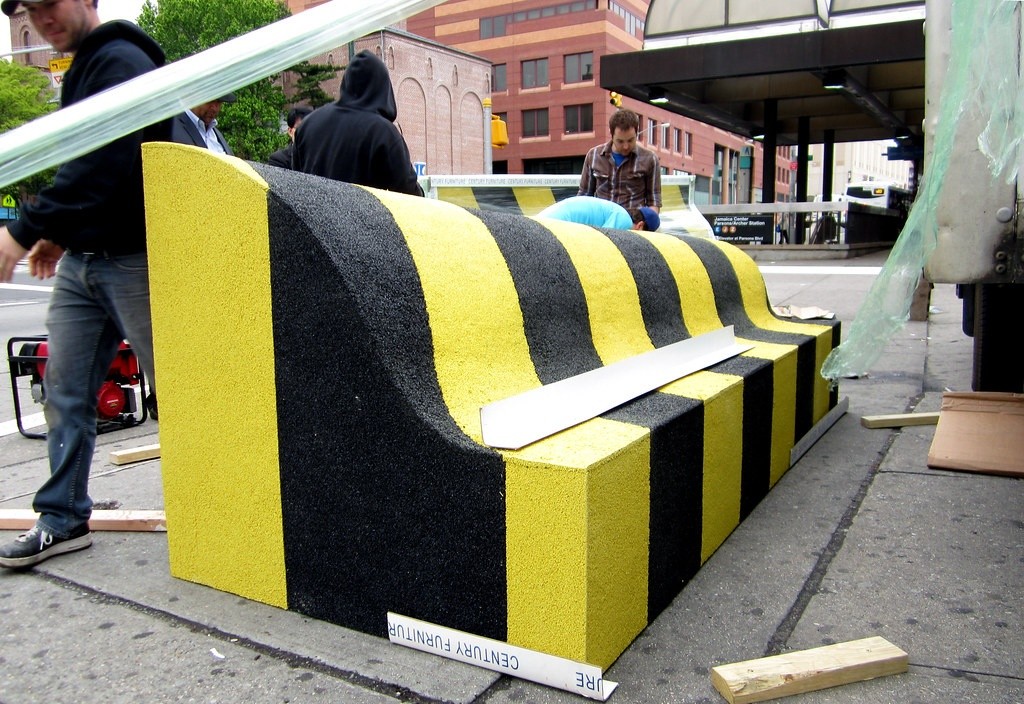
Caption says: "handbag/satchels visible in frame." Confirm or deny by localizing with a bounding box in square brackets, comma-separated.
[776, 224, 781, 232]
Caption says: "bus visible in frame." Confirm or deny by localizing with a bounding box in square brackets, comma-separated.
[842, 183, 913, 226]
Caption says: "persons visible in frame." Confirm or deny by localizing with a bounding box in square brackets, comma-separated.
[172, 92, 238, 155]
[776, 215, 791, 245]
[577, 109, 662, 214]
[266, 107, 316, 171]
[532, 195, 660, 232]
[0, 0, 166, 571]
[292, 48, 425, 208]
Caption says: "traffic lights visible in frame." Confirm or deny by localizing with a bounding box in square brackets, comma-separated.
[611, 91, 623, 108]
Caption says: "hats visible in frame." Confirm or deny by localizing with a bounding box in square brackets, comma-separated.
[639, 207, 661, 233]
[216, 92, 237, 103]
[1, 0, 43, 17]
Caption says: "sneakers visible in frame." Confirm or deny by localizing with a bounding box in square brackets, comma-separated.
[0, 522, 92, 567]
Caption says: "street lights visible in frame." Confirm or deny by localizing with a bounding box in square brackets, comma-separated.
[732, 139, 753, 203]
